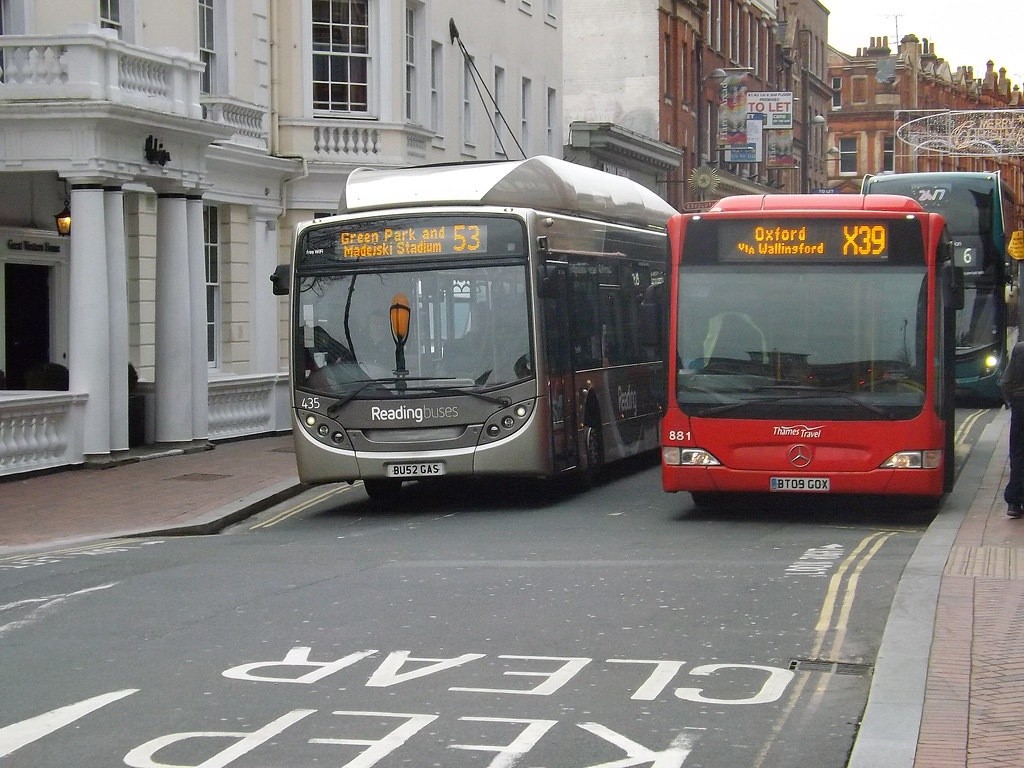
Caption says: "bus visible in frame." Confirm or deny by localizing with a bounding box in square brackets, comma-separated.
[286, 154, 668, 514]
[858, 172, 1024, 412]
[662, 194, 966, 524]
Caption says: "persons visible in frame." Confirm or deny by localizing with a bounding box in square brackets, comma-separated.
[336, 310, 396, 369]
[1000, 340, 1024, 516]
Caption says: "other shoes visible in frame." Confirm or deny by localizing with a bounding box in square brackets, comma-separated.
[1007, 503, 1024, 517]
